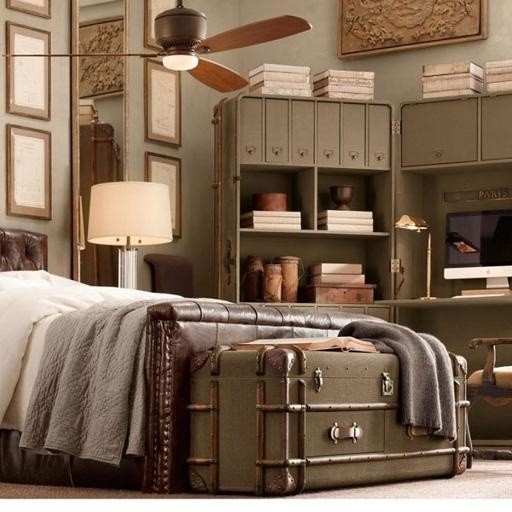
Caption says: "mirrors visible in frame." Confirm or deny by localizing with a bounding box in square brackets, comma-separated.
[71, 1, 130, 290]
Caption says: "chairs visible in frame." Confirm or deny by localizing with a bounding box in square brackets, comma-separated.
[143, 253, 194, 296]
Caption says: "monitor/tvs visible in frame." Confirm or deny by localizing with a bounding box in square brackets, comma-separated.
[442, 209, 512, 291]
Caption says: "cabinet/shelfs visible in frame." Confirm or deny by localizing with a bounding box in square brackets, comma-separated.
[212, 91, 395, 325]
[397, 91, 512, 307]
[81, 123, 122, 287]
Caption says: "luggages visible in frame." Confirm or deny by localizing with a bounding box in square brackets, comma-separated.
[186, 343, 470, 495]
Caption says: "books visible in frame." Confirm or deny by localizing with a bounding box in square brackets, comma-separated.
[485, 60, 512, 92]
[420, 62, 484, 99]
[318, 208, 375, 234]
[237, 336, 379, 353]
[242, 210, 304, 231]
[246, 62, 312, 99]
[316, 68, 375, 103]
[309, 261, 375, 301]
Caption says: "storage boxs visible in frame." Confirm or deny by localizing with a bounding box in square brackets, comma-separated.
[304, 282, 377, 304]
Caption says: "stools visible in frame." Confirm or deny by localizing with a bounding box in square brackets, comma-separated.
[464, 337, 511, 469]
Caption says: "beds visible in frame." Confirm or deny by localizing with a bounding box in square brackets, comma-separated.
[0, 228, 393, 494]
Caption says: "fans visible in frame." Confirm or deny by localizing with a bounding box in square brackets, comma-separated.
[0, 0, 312, 92]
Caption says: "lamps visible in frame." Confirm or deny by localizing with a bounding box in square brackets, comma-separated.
[86, 181, 175, 290]
[449, 232, 480, 255]
[161, 55, 200, 72]
[394, 214, 438, 300]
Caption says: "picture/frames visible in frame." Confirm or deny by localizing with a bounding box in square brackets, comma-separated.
[79, 15, 124, 100]
[336, 0, 488, 61]
[5, 21, 52, 122]
[143, 151, 182, 238]
[6, 124, 53, 220]
[144, 59, 182, 149]
[6, 1, 52, 19]
[143, 1, 176, 52]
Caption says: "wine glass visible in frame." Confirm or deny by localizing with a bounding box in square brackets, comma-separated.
[328, 184, 355, 211]
